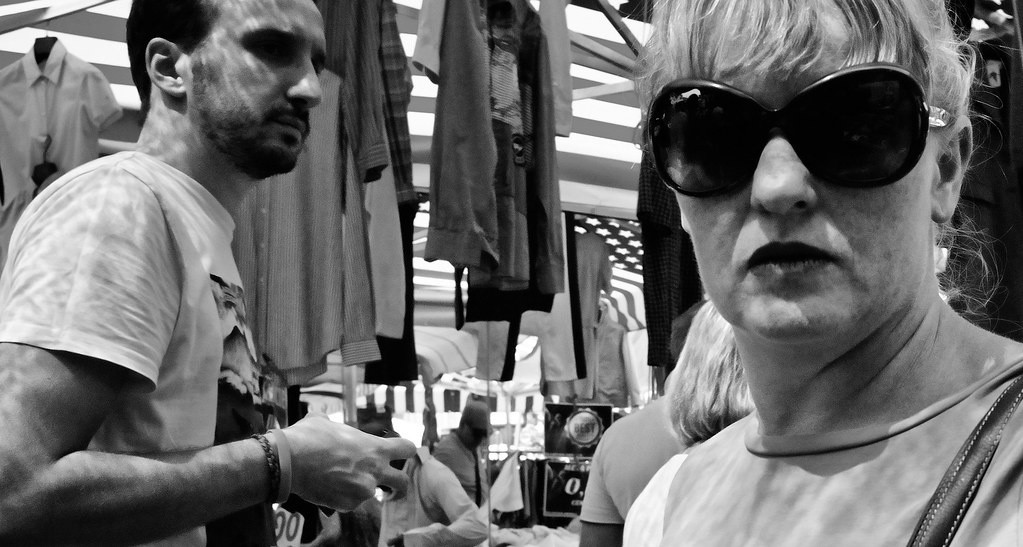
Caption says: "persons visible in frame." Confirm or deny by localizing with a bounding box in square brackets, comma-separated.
[358, 299, 754, 547]
[623, 0, 1023, 547]
[0, 1, 417, 547]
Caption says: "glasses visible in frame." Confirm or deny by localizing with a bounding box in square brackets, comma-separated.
[640, 58, 954, 199]
[465, 421, 487, 439]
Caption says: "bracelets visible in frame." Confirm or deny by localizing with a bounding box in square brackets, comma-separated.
[251, 428, 293, 503]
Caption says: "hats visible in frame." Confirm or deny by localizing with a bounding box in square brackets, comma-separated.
[464, 402, 490, 430]
